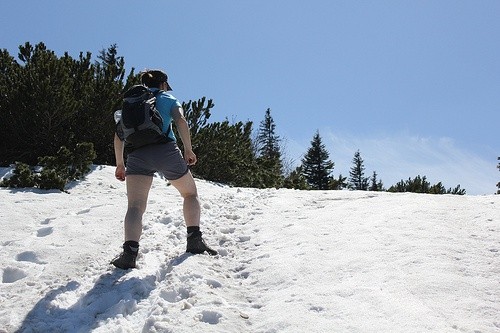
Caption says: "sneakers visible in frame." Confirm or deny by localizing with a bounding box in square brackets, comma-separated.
[186, 231, 217, 255]
[113, 245, 139, 269]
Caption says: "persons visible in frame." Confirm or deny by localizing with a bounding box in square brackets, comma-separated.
[109, 70, 218, 269]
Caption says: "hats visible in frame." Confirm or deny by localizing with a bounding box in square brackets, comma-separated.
[142, 70, 173, 91]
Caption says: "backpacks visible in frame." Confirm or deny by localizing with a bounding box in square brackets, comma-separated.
[113, 85, 174, 157]
